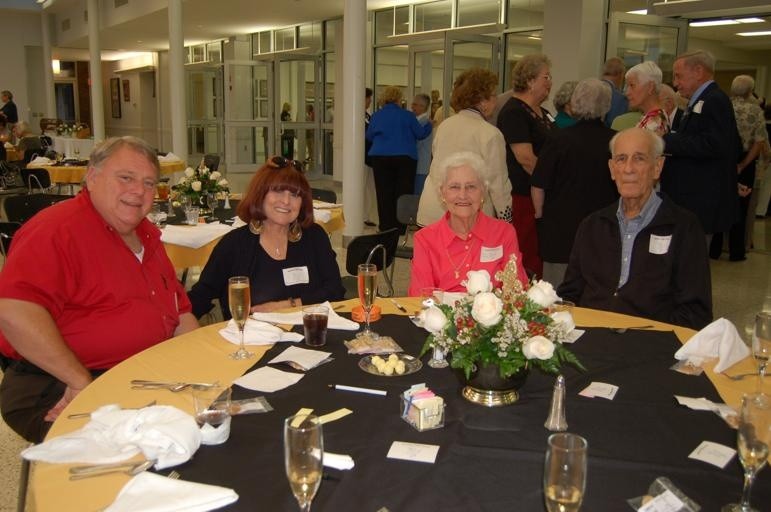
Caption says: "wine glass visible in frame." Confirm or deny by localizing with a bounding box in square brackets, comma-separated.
[280, 413, 325, 512]
[227, 275, 255, 360]
[719, 391, 770, 512]
[540, 432, 589, 512]
[354, 264, 379, 339]
[150, 203, 161, 230]
[181, 195, 193, 223]
[743, 310, 770, 409]
[206, 192, 221, 223]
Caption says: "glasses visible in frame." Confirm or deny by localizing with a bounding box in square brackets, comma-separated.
[266, 156, 305, 172]
[540, 73, 553, 78]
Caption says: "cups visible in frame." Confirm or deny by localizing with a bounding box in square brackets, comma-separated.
[419, 287, 445, 309]
[301, 304, 330, 347]
[186, 208, 200, 226]
[548, 300, 575, 338]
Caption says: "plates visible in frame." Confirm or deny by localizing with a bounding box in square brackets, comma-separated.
[358, 351, 423, 377]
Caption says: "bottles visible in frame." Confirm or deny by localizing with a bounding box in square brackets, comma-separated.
[542, 372, 571, 431]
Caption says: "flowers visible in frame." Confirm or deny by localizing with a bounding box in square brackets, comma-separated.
[56, 123, 70, 132]
[168, 164, 230, 199]
[68, 125, 82, 132]
[403, 269, 588, 380]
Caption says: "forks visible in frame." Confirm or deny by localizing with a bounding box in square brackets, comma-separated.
[166, 471, 180, 480]
[608, 325, 654, 335]
[722, 371, 771, 381]
[67, 399, 157, 419]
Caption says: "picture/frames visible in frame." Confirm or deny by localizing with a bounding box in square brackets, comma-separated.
[121, 79, 130, 102]
[109, 77, 121, 118]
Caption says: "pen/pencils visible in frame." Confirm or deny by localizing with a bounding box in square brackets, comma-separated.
[391, 299, 407, 313]
[327, 383, 388, 397]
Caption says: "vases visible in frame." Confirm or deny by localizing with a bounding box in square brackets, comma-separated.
[70, 131, 76, 137]
[449, 359, 529, 406]
[59, 131, 67, 136]
[187, 194, 212, 214]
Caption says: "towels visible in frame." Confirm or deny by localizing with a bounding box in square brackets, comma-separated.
[101, 471, 239, 512]
[157, 152, 180, 163]
[32, 156, 49, 164]
[249, 301, 360, 332]
[161, 225, 233, 249]
[20, 404, 201, 471]
[675, 317, 751, 373]
[312, 202, 343, 208]
[313, 208, 331, 224]
[218, 316, 303, 346]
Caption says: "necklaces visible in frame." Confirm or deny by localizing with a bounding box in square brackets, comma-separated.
[261, 230, 288, 259]
[444, 239, 478, 280]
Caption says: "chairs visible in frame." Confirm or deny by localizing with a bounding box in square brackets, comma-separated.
[388, 194, 421, 297]
[40, 137, 51, 150]
[20, 167, 52, 195]
[39, 118, 62, 129]
[191, 154, 221, 174]
[0, 221, 22, 266]
[0, 354, 30, 512]
[340, 228, 399, 301]
[0, 141, 20, 191]
[310, 187, 336, 204]
[2, 193, 74, 225]
[22, 148, 45, 168]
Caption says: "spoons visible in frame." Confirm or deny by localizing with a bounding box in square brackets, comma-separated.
[130, 383, 191, 393]
[68, 459, 157, 481]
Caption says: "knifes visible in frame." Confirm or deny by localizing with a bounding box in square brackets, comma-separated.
[130, 379, 220, 392]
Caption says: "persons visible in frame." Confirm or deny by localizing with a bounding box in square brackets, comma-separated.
[365, 86, 377, 227]
[746, 91, 771, 254]
[625, 61, 672, 137]
[1, 90, 42, 174]
[411, 92, 433, 195]
[553, 80, 581, 129]
[709, 73, 766, 262]
[656, 84, 687, 133]
[280, 102, 297, 137]
[434, 105, 456, 128]
[486, 87, 518, 125]
[496, 52, 561, 283]
[556, 129, 714, 332]
[531, 76, 623, 292]
[677, 91, 691, 110]
[0, 137, 202, 444]
[661, 48, 754, 256]
[600, 56, 629, 128]
[430, 89, 442, 120]
[366, 86, 438, 230]
[408, 151, 531, 296]
[306, 103, 316, 162]
[415, 68, 518, 229]
[187, 155, 350, 322]
